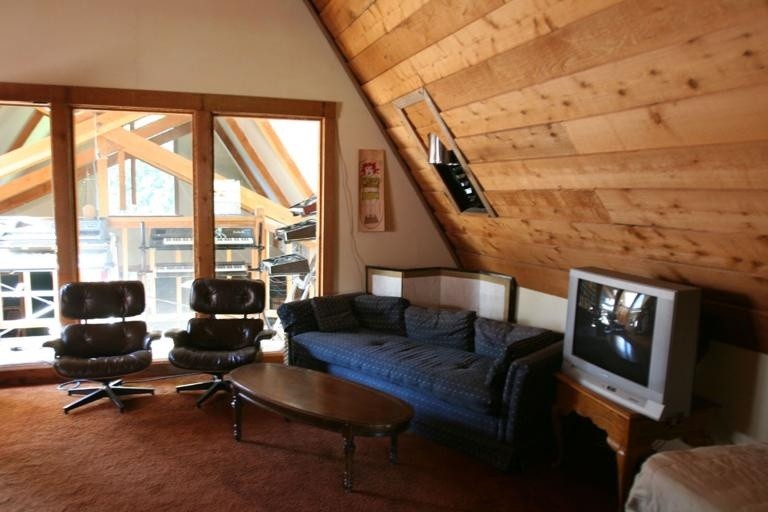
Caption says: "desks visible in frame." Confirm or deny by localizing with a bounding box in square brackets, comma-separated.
[624, 441, 768, 512]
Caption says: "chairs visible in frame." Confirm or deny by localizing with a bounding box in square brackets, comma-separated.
[42, 280, 155, 414]
[170, 278, 272, 409]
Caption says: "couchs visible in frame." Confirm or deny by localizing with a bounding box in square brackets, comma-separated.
[277, 292, 565, 475]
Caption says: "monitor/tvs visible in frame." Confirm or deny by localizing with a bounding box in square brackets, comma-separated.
[562, 266, 705, 422]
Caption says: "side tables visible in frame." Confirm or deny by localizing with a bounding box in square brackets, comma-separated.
[552, 371, 724, 511]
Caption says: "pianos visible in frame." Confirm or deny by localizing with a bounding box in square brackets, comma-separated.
[155, 261, 249, 276]
[149, 227, 256, 249]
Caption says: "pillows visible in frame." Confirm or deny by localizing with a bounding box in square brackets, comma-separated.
[311, 295, 355, 332]
[485, 331, 552, 407]
[277, 299, 318, 337]
[354, 295, 410, 338]
[405, 307, 476, 352]
[495, 323, 548, 362]
[473, 317, 513, 362]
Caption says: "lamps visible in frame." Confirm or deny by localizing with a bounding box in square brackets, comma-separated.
[428, 132, 449, 166]
